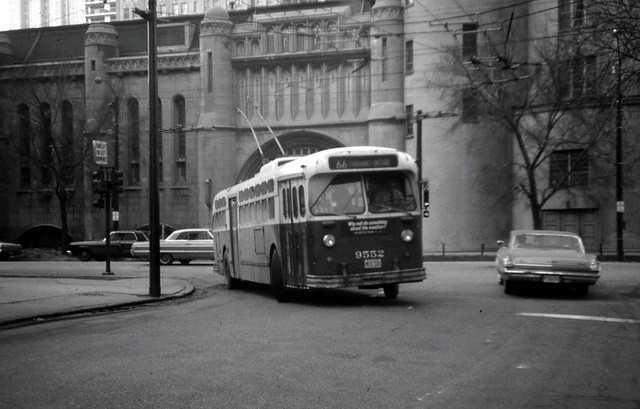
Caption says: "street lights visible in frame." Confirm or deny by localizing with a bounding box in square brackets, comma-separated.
[94, 76, 120, 168]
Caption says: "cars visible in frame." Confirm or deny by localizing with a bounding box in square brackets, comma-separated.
[131, 229, 215, 265]
[497, 230, 598, 296]
[0, 242, 23, 261]
[135, 223, 176, 239]
[66, 230, 148, 262]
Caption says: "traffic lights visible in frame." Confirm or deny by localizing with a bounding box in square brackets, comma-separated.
[111, 169, 123, 193]
[92, 169, 106, 208]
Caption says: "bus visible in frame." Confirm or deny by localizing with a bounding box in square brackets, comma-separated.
[212, 147, 425, 302]
